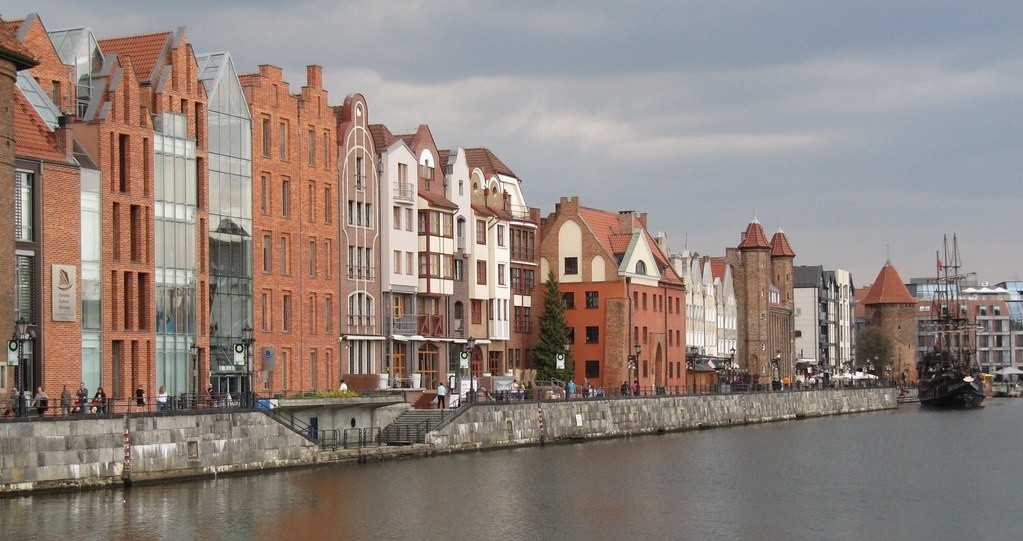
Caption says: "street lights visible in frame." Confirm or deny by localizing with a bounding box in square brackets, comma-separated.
[243, 324, 254, 409]
[12, 317, 36, 417]
[635, 344, 641, 397]
[730, 347, 736, 386]
[189, 343, 200, 408]
[690, 345, 698, 394]
[468, 336, 475, 403]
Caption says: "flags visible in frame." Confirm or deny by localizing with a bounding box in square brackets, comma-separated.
[938, 259, 943, 271]
[707, 360, 715, 368]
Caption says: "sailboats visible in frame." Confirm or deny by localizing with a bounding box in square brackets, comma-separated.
[917, 233, 986, 408]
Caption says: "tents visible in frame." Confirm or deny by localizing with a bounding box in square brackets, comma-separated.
[992, 367, 1023, 375]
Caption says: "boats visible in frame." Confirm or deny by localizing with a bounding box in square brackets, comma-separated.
[991, 366, 1023, 398]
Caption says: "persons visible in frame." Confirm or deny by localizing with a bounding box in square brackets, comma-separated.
[809, 376, 815, 390]
[796, 377, 803, 390]
[204, 390, 211, 407]
[581, 377, 590, 398]
[568, 379, 575, 394]
[60, 385, 71, 416]
[31, 386, 48, 417]
[437, 382, 446, 409]
[76, 382, 88, 413]
[156, 385, 167, 411]
[510, 380, 519, 400]
[803, 377, 810, 389]
[517, 381, 525, 400]
[621, 381, 629, 396]
[10, 387, 20, 417]
[92, 388, 107, 413]
[207, 384, 215, 407]
[632, 380, 637, 395]
[562, 382, 566, 400]
[597, 386, 605, 397]
[135, 384, 145, 412]
[339, 379, 347, 394]
[783, 376, 790, 390]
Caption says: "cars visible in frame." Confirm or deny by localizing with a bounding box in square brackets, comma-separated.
[536, 380, 565, 400]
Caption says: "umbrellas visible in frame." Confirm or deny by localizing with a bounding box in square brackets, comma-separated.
[831, 369, 879, 379]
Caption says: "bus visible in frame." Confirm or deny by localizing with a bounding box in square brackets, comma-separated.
[795, 362, 836, 389]
[685, 354, 731, 385]
